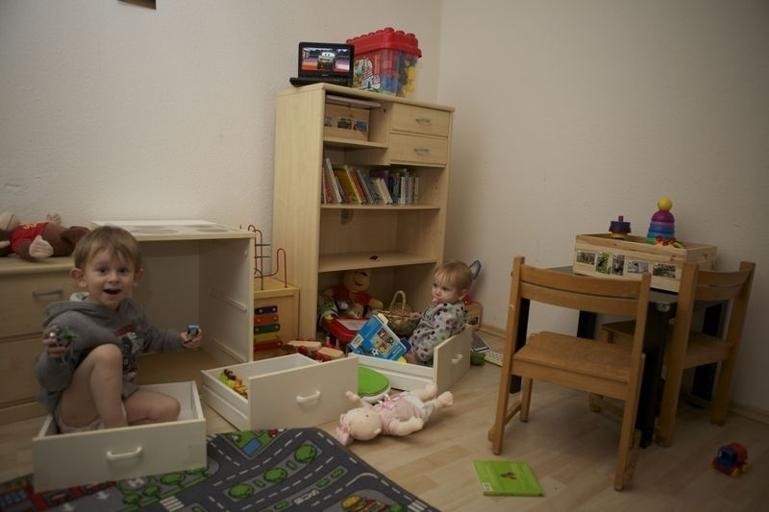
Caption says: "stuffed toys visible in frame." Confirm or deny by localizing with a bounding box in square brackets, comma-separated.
[323, 268, 384, 317]
[0, 210, 91, 264]
[709, 441, 750, 477]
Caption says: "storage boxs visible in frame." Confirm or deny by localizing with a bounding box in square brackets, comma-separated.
[346, 28, 423, 99]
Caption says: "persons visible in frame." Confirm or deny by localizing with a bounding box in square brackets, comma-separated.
[336, 379, 455, 446]
[31, 224, 204, 434]
[404, 260, 473, 367]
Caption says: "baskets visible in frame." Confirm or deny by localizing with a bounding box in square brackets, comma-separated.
[376, 289, 421, 335]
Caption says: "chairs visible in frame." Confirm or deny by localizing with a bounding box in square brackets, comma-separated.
[482, 252, 653, 494]
[589, 259, 760, 451]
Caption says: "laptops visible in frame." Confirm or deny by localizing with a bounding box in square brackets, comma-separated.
[289, 41, 354, 86]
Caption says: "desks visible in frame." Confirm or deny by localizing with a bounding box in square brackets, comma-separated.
[508, 263, 730, 451]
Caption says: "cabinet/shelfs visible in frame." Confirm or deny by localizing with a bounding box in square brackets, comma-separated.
[269, 80, 457, 344]
[1, 216, 257, 425]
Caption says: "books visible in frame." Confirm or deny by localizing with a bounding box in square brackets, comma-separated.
[471, 459, 545, 498]
[321, 157, 420, 204]
[346, 312, 411, 361]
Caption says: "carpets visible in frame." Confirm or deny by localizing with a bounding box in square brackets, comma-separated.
[1, 426, 442, 512]
